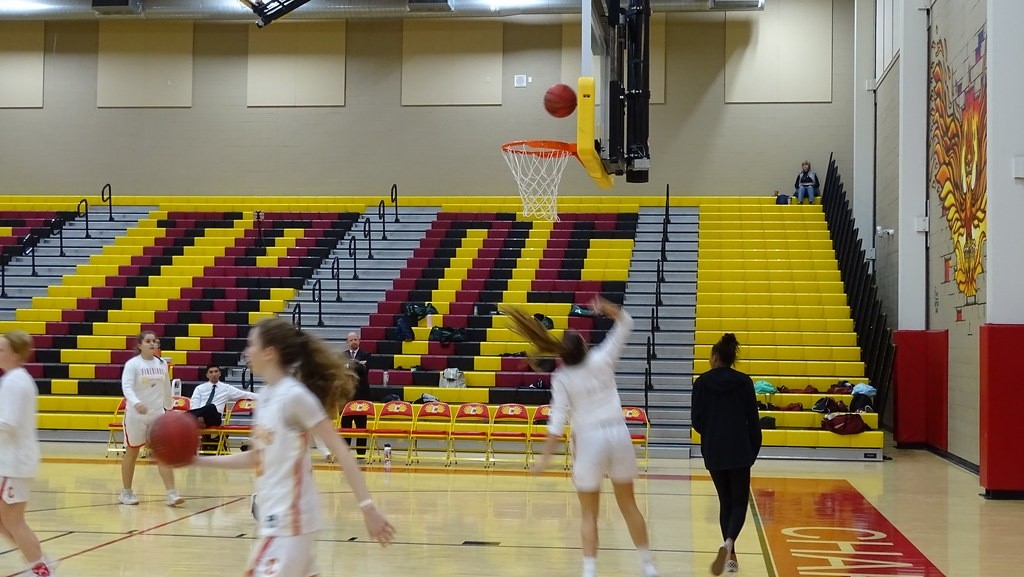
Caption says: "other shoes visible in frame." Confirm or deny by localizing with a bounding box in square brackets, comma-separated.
[641, 564, 659, 577]
[797, 201, 803, 205]
[810, 201, 816, 204]
[25, 557, 58, 577]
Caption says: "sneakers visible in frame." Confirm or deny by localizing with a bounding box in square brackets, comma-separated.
[119, 489, 138, 505]
[726, 560, 738, 572]
[166, 492, 184, 505]
[712, 543, 731, 575]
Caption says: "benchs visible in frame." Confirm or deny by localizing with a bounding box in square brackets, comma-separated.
[0, 194, 885, 446]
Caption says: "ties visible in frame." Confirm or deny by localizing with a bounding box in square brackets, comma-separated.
[205, 385, 216, 406]
[351, 351, 355, 359]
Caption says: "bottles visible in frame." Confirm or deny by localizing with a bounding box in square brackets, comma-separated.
[491, 311, 506, 315]
[473, 306, 479, 315]
[383, 367, 390, 387]
[384, 443, 392, 466]
[155, 339, 160, 358]
[426, 311, 432, 329]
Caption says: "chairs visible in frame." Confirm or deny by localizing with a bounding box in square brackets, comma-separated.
[330, 406, 339, 463]
[527, 404, 570, 472]
[370, 400, 419, 466]
[486, 404, 533, 471]
[170, 398, 189, 412]
[199, 402, 230, 456]
[448, 403, 496, 470]
[337, 400, 382, 464]
[218, 400, 257, 457]
[409, 402, 457, 466]
[621, 405, 652, 471]
[105, 400, 128, 457]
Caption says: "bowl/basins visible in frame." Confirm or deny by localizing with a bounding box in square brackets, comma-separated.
[164, 358, 172, 362]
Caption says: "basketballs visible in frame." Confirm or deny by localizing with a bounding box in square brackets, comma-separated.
[544, 84, 577, 118]
[151, 412, 200, 466]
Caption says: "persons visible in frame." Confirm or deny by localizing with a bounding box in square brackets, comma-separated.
[498, 296, 659, 576]
[0, 330, 55, 577]
[188, 363, 259, 427]
[793, 161, 821, 205]
[190, 320, 396, 577]
[118, 331, 185, 505]
[690, 332, 761, 576]
[338, 332, 372, 459]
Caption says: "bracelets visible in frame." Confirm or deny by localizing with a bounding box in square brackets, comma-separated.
[360, 499, 373, 507]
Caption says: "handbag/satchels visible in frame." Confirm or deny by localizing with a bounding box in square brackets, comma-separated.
[755, 379, 876, 436]
[440, 368, 467, 388]
[395, 317, 415, 342]
[405, 303, 438, 321]
[568, 304, 593, 316]
[428, 326, 471, 345]
[776, 195, 792, 205]
[541, 316, 554, 330]
